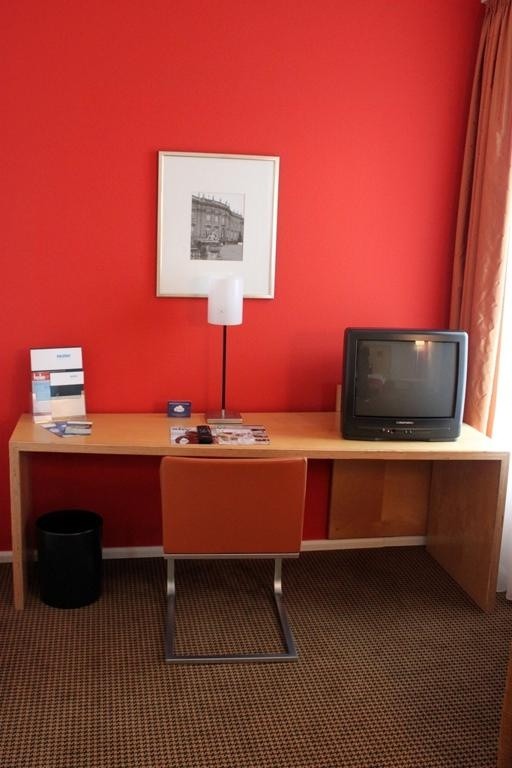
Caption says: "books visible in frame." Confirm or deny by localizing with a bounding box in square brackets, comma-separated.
[39, 420, 93, 439]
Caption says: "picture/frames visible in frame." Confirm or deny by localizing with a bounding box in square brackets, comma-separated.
[153, 149, 281, 301]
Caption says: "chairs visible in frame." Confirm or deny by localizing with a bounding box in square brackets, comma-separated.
[158, 454, 308, 664]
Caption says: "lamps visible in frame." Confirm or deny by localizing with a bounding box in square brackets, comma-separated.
[204, 275, 244, 425]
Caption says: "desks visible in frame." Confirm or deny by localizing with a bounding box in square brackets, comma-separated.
[8, 412, 510, 614]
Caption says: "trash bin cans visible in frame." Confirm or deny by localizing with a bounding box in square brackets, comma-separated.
[36, 510, 104, 609]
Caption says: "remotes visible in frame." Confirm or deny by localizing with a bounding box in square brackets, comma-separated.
[197, 425, 213, 443]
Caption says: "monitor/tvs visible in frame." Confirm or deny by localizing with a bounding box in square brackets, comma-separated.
[340, 327, 469, 442]
